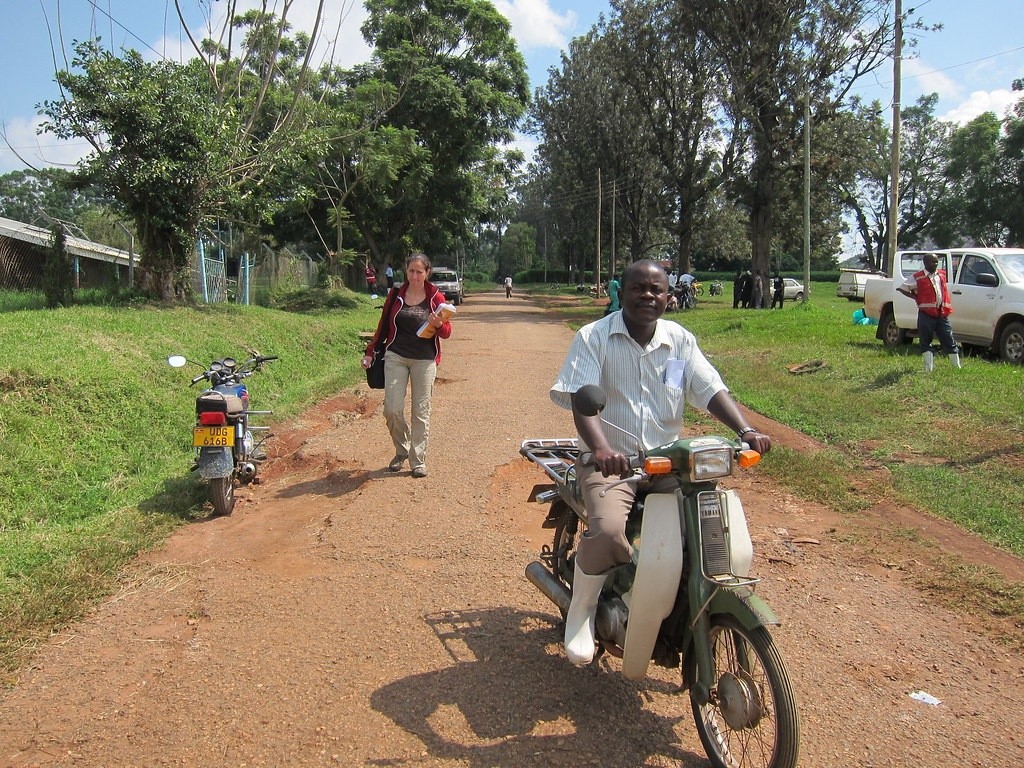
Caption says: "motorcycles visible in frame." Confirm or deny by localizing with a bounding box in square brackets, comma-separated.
[169, 353, 279, 515]
[587, 284, 607, 297]
[520, 384, 800, 768]
[666, 278, 704, 311]
[709, 279, 723, 297]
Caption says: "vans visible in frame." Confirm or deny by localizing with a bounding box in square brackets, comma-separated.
[837, 269, 886, 301]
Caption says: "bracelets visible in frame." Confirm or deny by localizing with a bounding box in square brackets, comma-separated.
[738, 427, 757, 437]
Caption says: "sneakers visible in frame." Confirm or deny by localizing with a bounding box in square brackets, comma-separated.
[389, 453, 409, 472]
[413, 467, 428, 477]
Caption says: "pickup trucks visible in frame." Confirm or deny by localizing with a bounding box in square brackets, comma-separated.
[863, 247, 1024, 365]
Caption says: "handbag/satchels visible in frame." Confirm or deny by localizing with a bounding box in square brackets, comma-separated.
[365, 342, 385, 390]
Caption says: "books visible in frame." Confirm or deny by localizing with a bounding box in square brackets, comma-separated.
[417, 303, 457, 338]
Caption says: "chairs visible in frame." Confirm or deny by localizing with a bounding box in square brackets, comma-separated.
[965, 261, 990, 285]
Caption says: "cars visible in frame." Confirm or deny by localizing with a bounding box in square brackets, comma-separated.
[769, 278, 812, 301]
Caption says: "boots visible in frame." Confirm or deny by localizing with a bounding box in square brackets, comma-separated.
[949, 353, 960, 370]
[564, 554, 608, 665]
[922, 351, 934, 372]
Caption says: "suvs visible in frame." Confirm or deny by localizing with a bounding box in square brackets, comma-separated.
[428, 270, 464, 305]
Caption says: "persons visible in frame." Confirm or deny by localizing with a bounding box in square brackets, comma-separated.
[899, 253, 961, 373]
[361, 253, 451, 478]
[733, 268, 768, 309]
[770, 271, 784, 309]
[503, 274, 513, 298]
[608, 273, 622, 314]
[385, 263, 394, 296]
[679, 269, 696, 309]
[576, 282, 585, 293]
[363, 261, 377, 296]
[664, 267, 678, 292]
[549, 259, 772, 664]
